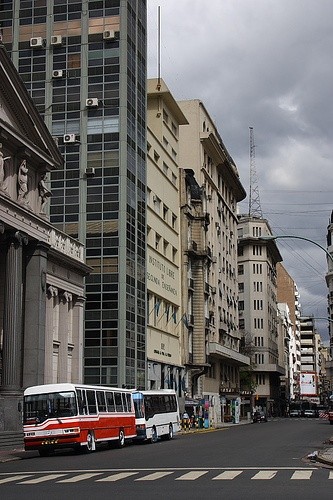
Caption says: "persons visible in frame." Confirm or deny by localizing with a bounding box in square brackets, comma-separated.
[182, 412, 195, 427]
[254, 409, 265, 423]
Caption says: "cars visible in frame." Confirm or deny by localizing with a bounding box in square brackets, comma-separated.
[252, 411, 267, 423]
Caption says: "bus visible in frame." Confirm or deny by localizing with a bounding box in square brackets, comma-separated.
[326, 394, 333, 425]
[289, 401, 320, 418]
[130, 388, 181, 444]
[17, 383, 139, 458]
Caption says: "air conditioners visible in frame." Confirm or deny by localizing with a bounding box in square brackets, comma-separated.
[86, 97, 98, 107]
[29, 37, 44, 48]
[52, 70, 63, 78]
[51, 35, 63, 46]
[63, 133, 76, 143]
[85, 167, 95, 174]
[103, 30, 115, 39]
